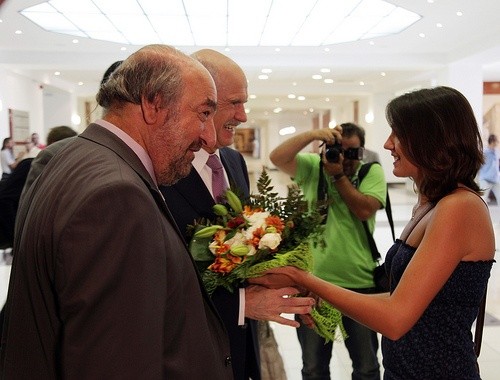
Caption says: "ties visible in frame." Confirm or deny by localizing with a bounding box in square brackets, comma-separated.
[205, 153, 228, 203]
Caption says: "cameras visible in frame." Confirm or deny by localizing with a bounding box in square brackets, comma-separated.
[324, 140, 342, 163]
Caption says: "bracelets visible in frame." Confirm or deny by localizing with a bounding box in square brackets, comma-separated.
[334, 173, 345, 180]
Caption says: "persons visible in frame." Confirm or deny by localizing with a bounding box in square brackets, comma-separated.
[269, 122, 386, 380]
[0, 61, 125, 250]
[478, 134, 500, 206]
[0, 43, 233, 380]
[248, 86, 500, 380]
[158, 49, 317, 380]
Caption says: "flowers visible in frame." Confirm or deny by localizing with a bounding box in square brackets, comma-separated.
[186, 165, 350, 346]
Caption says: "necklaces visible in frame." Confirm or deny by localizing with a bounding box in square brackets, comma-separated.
[411, 204, 423, 221]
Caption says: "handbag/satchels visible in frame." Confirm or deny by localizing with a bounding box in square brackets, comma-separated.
[372, 262, 388, 292]
